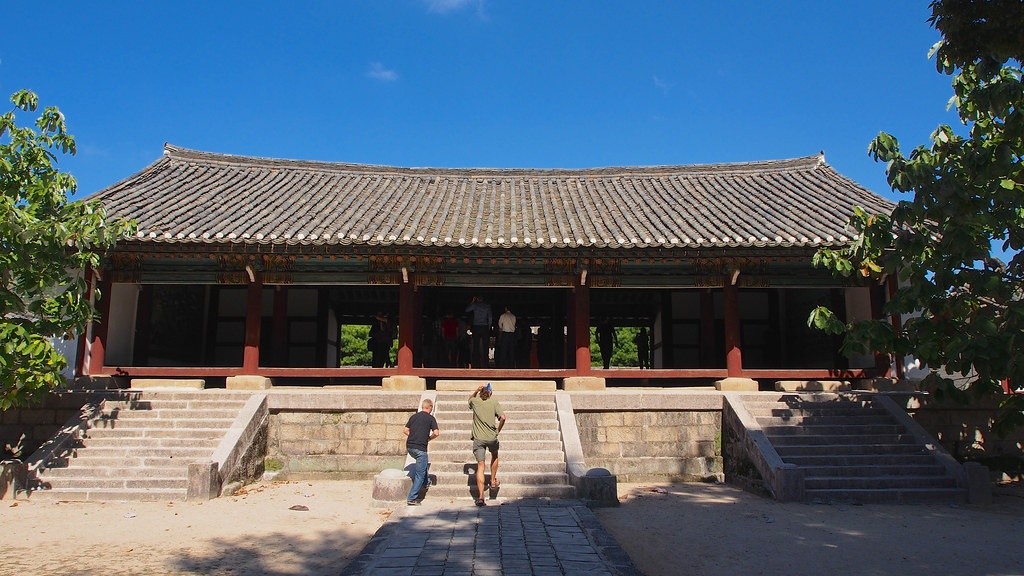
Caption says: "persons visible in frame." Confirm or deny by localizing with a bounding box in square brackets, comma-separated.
[368, 308, 394, 368]
[633, 328, 649, 369]
[421, 293, 564, 369]
[468, 384, 506, 505]
[403, 399, 439, 505]
[596, 315, 618, 369]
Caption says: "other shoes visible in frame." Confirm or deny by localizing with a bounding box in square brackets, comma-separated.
[407, 499, 417, 505]
[424, 480, 432, 490]
[490, 479, 500, 490]
[475, 499, 486, 506]
